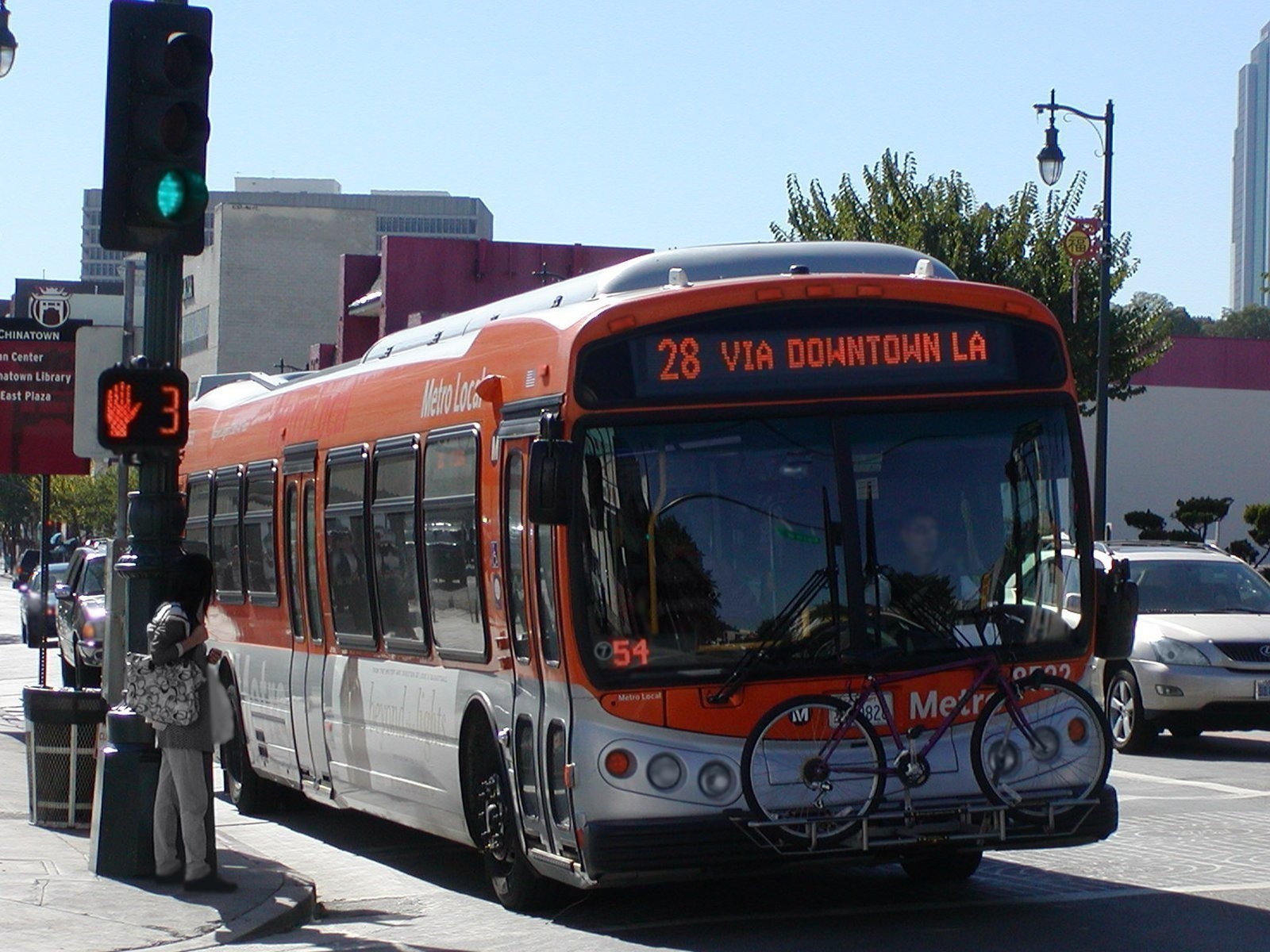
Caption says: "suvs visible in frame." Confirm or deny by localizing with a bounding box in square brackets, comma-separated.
[51, 536, 111, 687]
[12, 548, 40, 590]
[1003, 529, 1270, 753]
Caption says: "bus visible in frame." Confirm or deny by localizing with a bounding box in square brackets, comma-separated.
[171, 240, 1140, 918]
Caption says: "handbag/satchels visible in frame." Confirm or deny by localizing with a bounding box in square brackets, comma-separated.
[124, 605, 202, 725]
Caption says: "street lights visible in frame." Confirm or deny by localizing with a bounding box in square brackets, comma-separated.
[1032, 88, 1114, 544]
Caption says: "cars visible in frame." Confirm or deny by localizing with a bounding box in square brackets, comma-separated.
[19, 563, 73, 648]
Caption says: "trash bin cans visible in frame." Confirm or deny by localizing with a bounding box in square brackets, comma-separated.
[23, 684, 108, 830]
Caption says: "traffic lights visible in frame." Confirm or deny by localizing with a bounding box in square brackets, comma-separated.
[98, 0, 215, 256]
[96, 364, 189, 451]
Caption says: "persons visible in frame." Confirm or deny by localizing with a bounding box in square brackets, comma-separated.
[222, 529, 420, 641]
[146, 552, 240, 894]
[863, 511, 981, 623]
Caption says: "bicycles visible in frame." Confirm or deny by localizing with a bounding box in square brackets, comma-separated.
[739, 608, 1114, 847]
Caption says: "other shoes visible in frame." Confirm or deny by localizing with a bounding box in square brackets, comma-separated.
[157, 869, 185, 886]
[185, 872, 237, 893]
